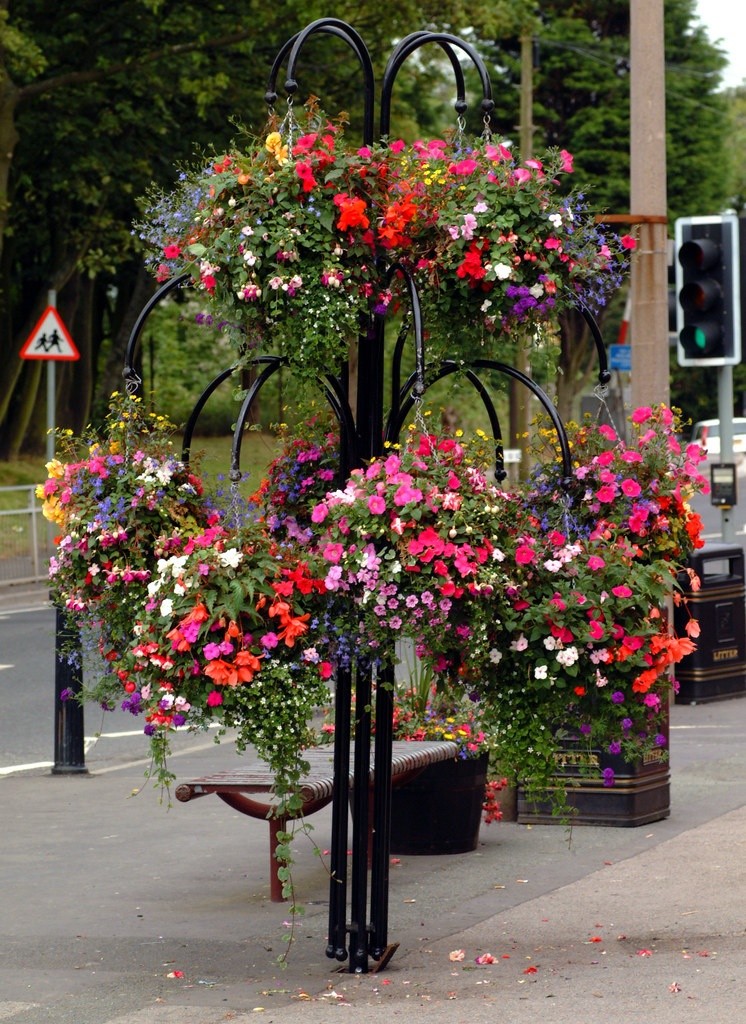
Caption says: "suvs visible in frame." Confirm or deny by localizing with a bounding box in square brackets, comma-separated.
[690, 417, 746, 458]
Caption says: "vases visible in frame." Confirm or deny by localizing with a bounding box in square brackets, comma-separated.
[371, 750, 489, 855]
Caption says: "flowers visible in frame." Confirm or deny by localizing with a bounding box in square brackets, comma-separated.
[32, 94, 713, 971]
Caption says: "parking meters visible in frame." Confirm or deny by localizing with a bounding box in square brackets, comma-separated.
[709, 463, 737, 509]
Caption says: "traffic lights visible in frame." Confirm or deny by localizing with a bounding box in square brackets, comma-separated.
[672, 212, 742, 367]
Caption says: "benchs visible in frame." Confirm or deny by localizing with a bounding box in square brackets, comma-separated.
[174, 736, 463, 904]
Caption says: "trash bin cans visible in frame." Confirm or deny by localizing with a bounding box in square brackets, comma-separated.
[672, 543, 746, 705]
